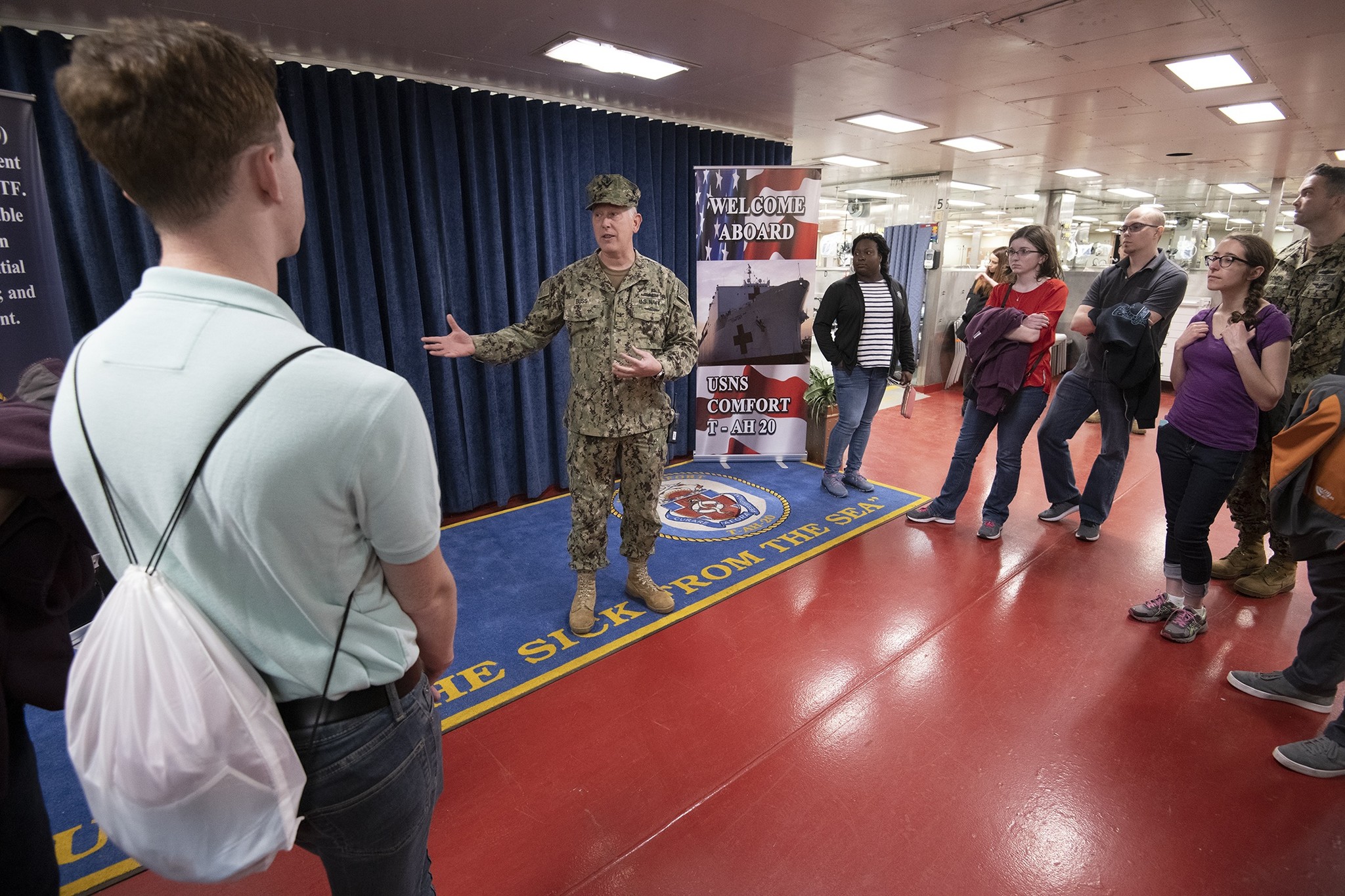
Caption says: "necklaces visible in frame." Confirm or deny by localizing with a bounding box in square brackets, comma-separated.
[1013, 281, 1037, 301]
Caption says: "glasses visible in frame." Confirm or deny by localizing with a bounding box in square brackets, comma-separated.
[1204, 255, 1259, 269]
[1005, 248, 1042, 257]
[1117, 222, 1159, 234]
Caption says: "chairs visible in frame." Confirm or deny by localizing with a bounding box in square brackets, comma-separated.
[1050, 333, 1067, 377]
[944, 311, 970, 390]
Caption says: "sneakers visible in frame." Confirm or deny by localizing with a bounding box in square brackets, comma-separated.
[1225, 668, 1336, 714]
[1038, 494, 1083, 522]
[1160, 604, 1208, 642]
[905, 507, 956, 523]
[569, 571, 597, 635]
[821, 471, 848, 497]
[1272, 735, 1345, 777]
[1128, 589, 1184, 624]
[1075, 520, 1100, 541]
[977, 520, 1003, 540]
[1234, 554, 1296, 598]
[625, 559, 675, 612]
[1210, 532, 1267, 579]
[843, 469, 874, 492]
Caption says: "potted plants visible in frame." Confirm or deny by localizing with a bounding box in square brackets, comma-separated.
[803, 365, 844, 465]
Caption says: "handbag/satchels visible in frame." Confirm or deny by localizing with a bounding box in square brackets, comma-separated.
[960, 365, 1027, 415]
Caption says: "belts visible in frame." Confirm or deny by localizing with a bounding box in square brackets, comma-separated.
[279, 672, 410, 728]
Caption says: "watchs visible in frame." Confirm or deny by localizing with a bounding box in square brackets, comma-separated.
[652, 367, 665, 381]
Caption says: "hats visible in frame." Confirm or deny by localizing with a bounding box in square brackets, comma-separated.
[584, 172, 642, 209]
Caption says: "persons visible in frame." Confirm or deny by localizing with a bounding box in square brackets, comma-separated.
[1130, 234, 1298, 638]
[961, 247, 1009, 417]
[1040, 206, 1188, 539]
[812, 232, 915, 497]
[50, 9, 457, 896]
[422, 175, 700, 634]
[1227, 341, 1345, 779]
[1211, 164, 1345, 597]
[906, 225, 1070, 538]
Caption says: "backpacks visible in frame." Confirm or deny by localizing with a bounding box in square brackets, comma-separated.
[64, 323, 380, 883]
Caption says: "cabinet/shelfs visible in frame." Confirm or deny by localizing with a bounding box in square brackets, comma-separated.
[1161, 297, 1211, 382]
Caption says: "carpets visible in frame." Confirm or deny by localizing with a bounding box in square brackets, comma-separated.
[25, 458, 934, 896]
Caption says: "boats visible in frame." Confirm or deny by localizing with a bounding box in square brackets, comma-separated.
[698, 263, 811, 366]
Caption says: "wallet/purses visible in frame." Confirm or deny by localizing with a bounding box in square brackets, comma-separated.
[900, 383, 916, 418]
